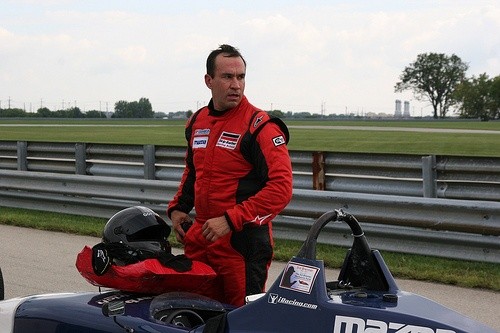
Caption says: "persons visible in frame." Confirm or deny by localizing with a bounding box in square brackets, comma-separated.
[167, 46, 292, 309]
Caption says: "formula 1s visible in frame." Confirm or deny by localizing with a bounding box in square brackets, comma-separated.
[0, 208, 500, 333]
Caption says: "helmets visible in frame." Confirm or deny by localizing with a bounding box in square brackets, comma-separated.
[102, 205, 172, 266]
